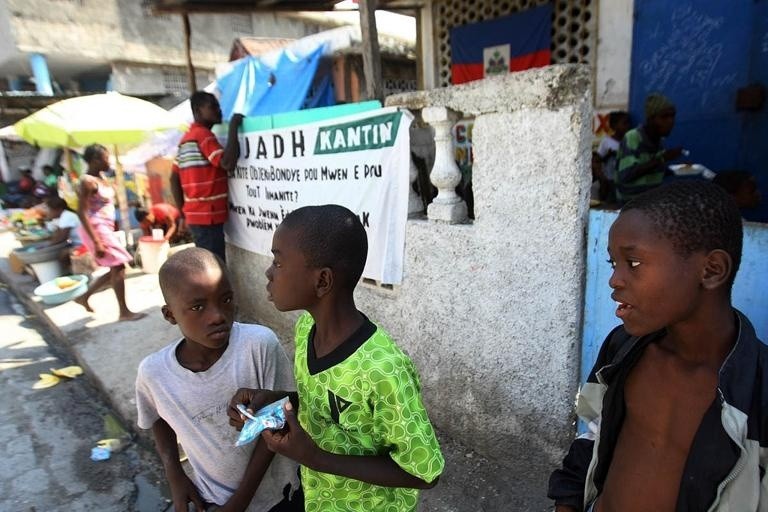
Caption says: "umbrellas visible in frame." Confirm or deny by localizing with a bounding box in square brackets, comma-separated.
[0, 123, 76, 172]
[8, 89, 183, 231]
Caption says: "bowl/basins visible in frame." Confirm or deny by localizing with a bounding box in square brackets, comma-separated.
[33, 273, 90, 305]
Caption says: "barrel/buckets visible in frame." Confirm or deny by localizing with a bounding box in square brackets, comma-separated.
[29, 258, 64, 284]
[138, 236, 170, 274]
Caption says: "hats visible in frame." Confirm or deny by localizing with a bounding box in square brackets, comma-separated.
[646, 92, 674, 118]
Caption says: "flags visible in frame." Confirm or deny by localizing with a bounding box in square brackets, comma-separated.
[447, 4, 558, 91]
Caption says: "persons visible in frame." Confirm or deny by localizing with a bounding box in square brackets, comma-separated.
[68, 142, 149, 324]
[612, 93, 692, 203]
[711, 169, 764, 224]
[131, 245, 304, 509]
[224, 201, 451, 510]
[170, 90, 248, 265]
[596, 110, 637, 203]
[545, 179, 768, 511]
[592, 152, 616, 204]
[0, 161, 193, 277]
